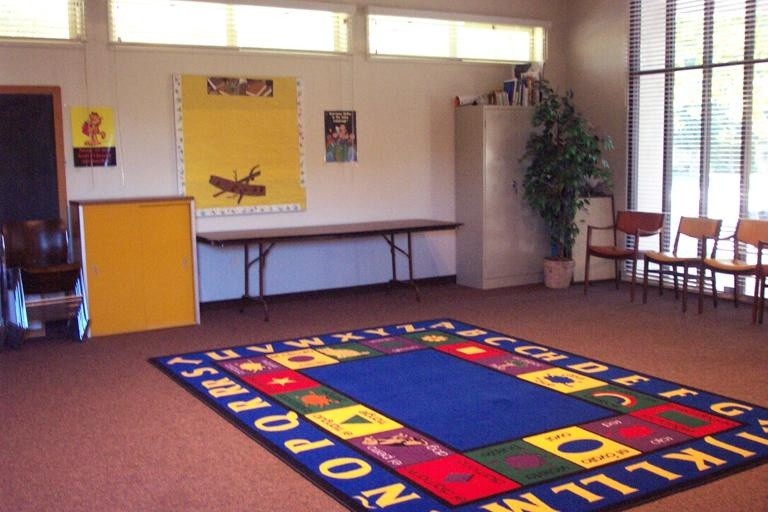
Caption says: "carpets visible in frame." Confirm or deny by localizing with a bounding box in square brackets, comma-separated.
[147, 317, 768, 512]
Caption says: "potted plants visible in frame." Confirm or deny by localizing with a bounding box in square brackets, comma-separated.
[519, 81, 615, 290]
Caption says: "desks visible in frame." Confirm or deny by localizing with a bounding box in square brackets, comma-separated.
[196, 219, 463, 322]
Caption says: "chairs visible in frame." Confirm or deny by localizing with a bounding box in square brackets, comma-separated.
[0, 218, 91, 350]
[584, 211, 768, 324]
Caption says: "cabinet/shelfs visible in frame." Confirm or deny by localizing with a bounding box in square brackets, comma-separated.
[69, 196, 202, 339]
[456, 105, 560, 290]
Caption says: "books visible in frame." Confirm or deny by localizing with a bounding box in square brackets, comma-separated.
[488, 73, 545, 107]
